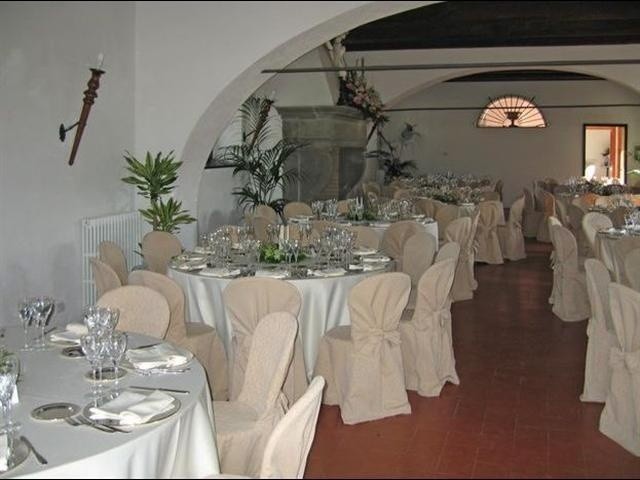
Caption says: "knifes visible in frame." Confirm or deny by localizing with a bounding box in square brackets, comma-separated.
[129, 386, 190, 395]
[600, 232, 622, 235]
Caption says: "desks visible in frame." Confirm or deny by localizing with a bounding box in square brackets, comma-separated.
[0, 323, 223, 480]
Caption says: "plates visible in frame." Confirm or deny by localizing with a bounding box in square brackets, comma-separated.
[117, 347, 195, 372]
[83, 390, 181, 425]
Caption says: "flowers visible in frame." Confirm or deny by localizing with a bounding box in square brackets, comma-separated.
[338, 66, 384, 124]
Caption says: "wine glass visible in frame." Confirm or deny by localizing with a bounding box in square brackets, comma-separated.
[199, 223, 356, 272]
[0, 296, 127, 473]
[312, 193, 414, 222]
[624, 206, 640, 234]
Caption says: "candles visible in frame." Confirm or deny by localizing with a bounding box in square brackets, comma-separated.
[96, 51, 105, 69]
